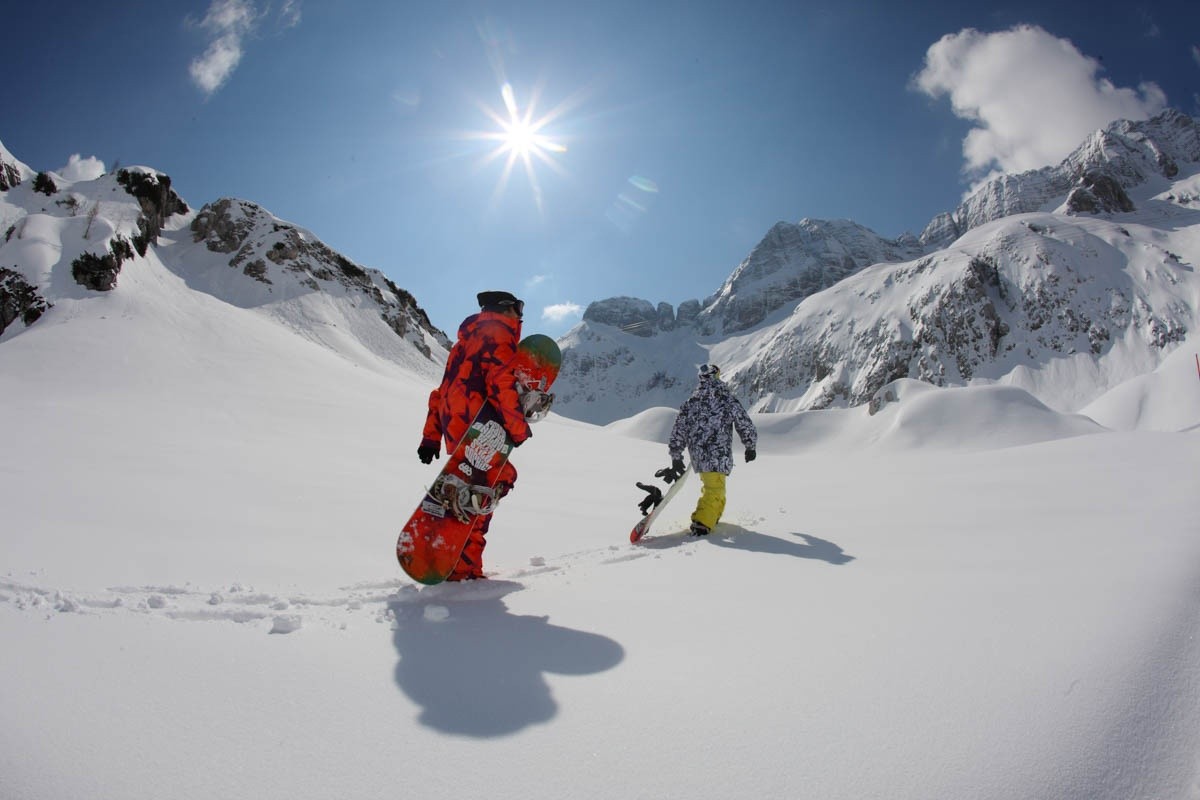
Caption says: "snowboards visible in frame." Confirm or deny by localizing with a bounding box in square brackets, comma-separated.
[629, 462, 693, 544]
[395, 333, 563, 586]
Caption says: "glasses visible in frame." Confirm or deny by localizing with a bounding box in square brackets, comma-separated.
[715, 371, 719, 379]
[514, 300, 524, 315]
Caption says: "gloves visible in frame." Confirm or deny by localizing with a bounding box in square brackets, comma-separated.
[744, 450, 756, 463]
[515, 438, 526, 447]
[673, 459, 686, 474]
[418, 440, 441, 464]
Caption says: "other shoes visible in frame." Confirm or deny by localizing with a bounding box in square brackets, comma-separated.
[690, 522, 710, 536]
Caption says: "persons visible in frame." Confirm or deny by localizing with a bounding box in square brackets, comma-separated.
[668, 364, 757, 536]
[417, 291, 532, 583]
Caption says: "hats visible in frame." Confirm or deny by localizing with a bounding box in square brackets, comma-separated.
[477, 291, 517, 311]
